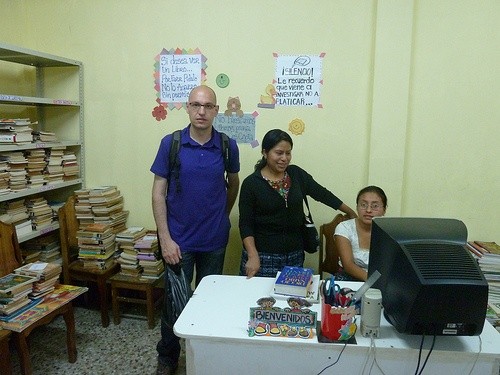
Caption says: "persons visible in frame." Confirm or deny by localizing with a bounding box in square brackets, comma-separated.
[150, 84, 240, 374]
[332, 186, 387, 281]
[236, 129, 357, 279]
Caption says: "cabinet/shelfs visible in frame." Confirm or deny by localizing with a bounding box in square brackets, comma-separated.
[0, 43, 86, 268]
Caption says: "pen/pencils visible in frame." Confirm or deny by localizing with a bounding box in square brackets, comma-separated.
[345, 294, 356, 307]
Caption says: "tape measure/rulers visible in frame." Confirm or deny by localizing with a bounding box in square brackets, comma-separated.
[352, 269, 381, 303]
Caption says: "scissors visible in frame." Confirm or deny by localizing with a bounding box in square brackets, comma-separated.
[323, 277, 341, 297]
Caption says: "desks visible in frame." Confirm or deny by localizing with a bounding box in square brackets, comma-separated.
[174, 273, 500, 375]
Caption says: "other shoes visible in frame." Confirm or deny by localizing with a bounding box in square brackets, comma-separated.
[156, 362, 178, 374]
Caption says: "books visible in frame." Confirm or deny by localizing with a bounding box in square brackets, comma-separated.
[0, 118, 163, 328]
[272, 265, 320, 304]
[466, 241, 500, 302]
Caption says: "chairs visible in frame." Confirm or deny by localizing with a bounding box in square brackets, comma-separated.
[320, 212, 357, 279]
[0, 193, 120, 371]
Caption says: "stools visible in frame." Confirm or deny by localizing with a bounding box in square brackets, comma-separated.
[107, 272, 164, 330]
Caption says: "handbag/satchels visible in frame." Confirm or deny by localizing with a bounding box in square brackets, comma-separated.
[303, 214, 320, 254]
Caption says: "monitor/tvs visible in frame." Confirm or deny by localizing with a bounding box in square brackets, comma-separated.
[367, 216, 489, 337]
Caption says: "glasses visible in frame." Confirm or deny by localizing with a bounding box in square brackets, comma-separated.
[190, 103, 214, 111]
[357, 202, 382, 211]
